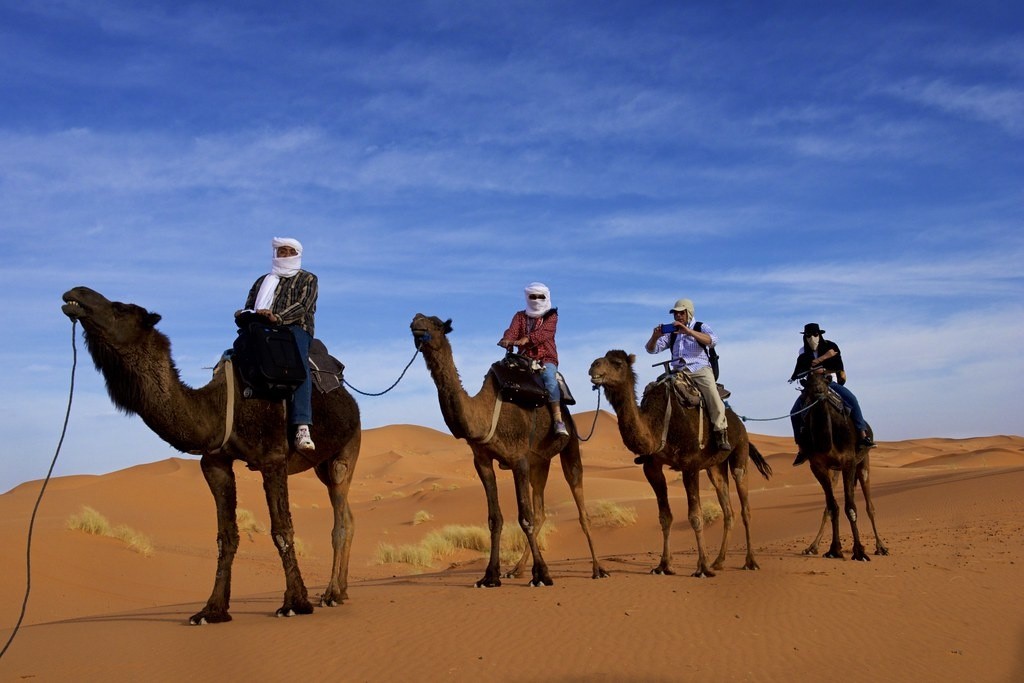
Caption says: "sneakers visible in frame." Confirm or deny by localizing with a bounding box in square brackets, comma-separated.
[717, 429, 731, 451]
[634, 456, 644, 464]
[554, 421, 568, 436]
[295, 429, 315, 451]
[859, 436, 878, 450]
[793, 450, 807, 466]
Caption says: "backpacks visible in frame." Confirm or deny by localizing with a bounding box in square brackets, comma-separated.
[669, 322, 719, 382]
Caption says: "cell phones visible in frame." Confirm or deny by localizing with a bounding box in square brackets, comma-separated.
[661, 323, 678, 334]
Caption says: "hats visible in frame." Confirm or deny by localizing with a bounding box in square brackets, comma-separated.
[800, 323, 826, 334]
[669, 299, 694, 324]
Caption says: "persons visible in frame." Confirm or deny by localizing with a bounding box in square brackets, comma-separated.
[212, 237, 318, 450]
[633, 299, 729, 464]
[498, 282, 570, 470]
[785, 322, 878, 466]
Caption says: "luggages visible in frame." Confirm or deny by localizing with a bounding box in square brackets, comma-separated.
[231, 312, 307, 400]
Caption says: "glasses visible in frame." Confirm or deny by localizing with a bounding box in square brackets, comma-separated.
[529, 294, 545, 300]
[805, 333, 819, 338]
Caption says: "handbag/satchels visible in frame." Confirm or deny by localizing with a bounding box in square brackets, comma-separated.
[491, 353, 550, 406]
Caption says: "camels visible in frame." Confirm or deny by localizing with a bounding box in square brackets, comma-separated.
[788, 365, 891, 563]
[63, 285, 362, 627]
[589, 350, 772, 578]
[411, 311, 613, 591]
